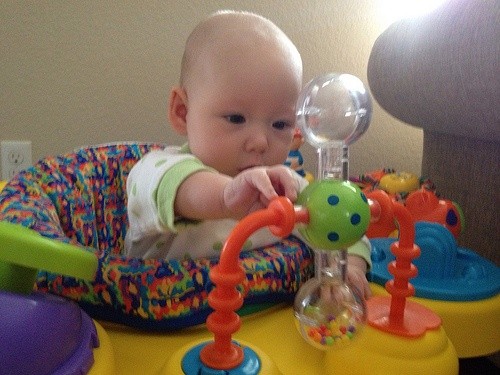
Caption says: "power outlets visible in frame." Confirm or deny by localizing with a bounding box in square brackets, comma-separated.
[0, 141, 34, 183]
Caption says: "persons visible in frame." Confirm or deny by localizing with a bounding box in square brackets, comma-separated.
[123, 10, 373, 302]
